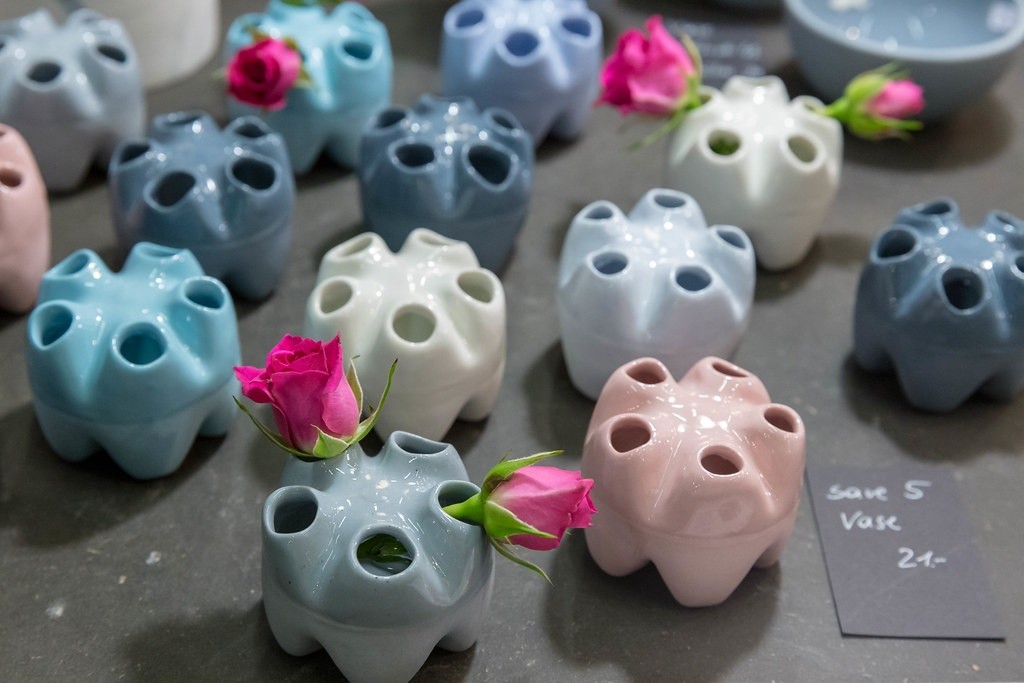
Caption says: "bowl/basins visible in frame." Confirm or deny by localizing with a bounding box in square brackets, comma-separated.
[783, 1, 1024, 125]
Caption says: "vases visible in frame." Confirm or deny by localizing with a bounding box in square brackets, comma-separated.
[293, 224, 516, 440]
[0, 0, 148, 194]
[662, 77, 851, 273]
[0, 122, 53, 313]
[581, 347, 811, 609]
[849, 198, 1024, 420]
[435, 0, 610, 143]
[555, 183, 767, 405]
[354, 91, 537, 277]
[221, 0, 396, 176]
[253, 407, 511, 683]
[108, 106, 299, 302]
[22, 240, 246, 480]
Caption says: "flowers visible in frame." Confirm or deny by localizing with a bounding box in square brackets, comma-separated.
[209, 21, 317, 115]
[229, 332, 605, 587]
[588, 11, 931, 157]
